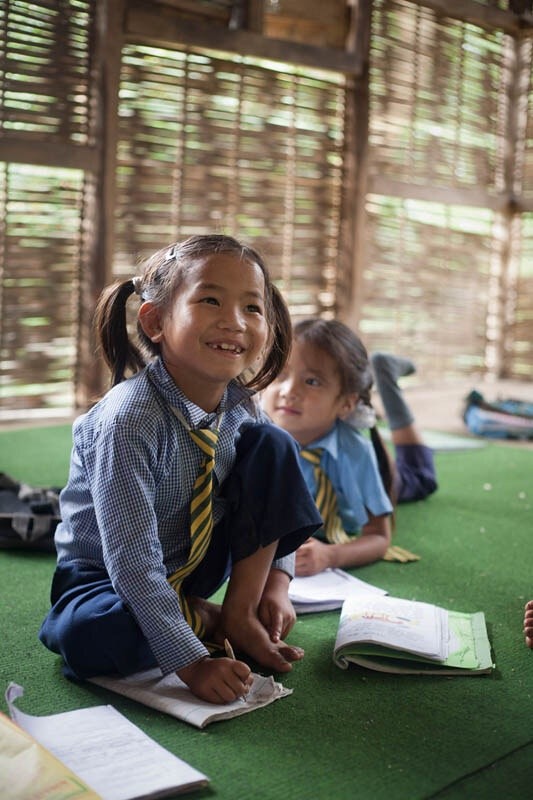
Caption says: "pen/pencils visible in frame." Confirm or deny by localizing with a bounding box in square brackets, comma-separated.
[224, 638, 246, 702]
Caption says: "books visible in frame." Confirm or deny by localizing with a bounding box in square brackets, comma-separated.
[332, 596, 493, 675]
[289, 567, 389, 614]
[86, 665, 294, 729]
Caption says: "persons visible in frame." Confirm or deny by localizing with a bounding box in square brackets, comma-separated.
[258, 318, 438, 580]
[40, 232, 324, 706]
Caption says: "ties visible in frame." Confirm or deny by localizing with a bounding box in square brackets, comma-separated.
[300, 448, 421, 563]
[166, 408, 225, 640]
